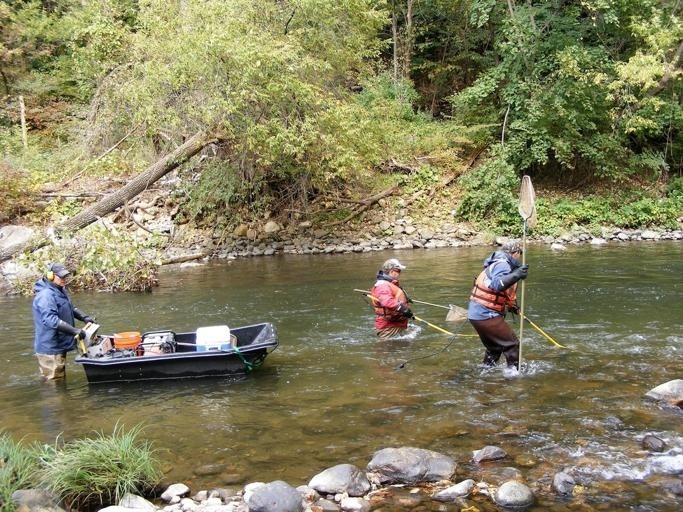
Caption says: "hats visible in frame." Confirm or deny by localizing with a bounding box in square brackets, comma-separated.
[503, 240, 523, 254]
[382, 258, 406, 271]
[52, 264, 70, 278]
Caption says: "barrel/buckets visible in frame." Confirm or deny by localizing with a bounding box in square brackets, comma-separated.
[114, 331, 141, 355]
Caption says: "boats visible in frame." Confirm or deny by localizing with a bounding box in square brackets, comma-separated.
[75, 317, 279, 383]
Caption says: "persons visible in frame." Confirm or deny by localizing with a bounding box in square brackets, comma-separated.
[371, 258, 413, 341]
[465, 240, 530, 374]
[31, 264, 98, 384]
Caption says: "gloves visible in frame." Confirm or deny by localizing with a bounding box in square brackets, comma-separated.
[500, 265, 528, 287]
[74, 308, 98, 324]
[508, 300, 520, 315]
[58, 320, 86, 339]
[407, 296, 414, 304]
[399, 304, 415, 320]
